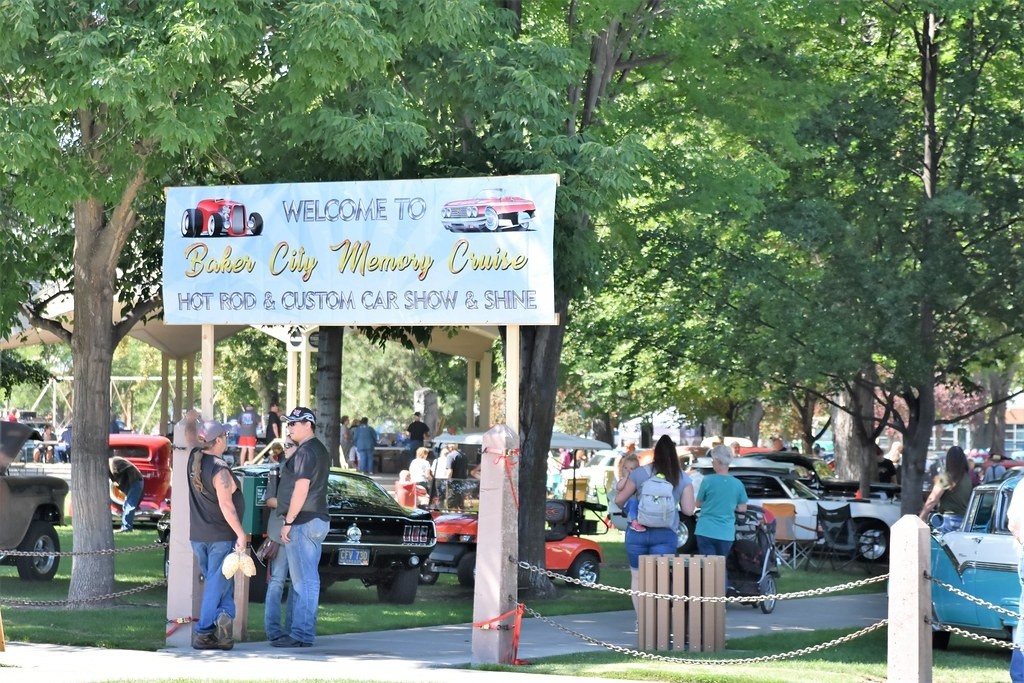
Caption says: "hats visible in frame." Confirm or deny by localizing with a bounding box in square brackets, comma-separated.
[197, 421, 232, 443]
[280, 406, 316, 427]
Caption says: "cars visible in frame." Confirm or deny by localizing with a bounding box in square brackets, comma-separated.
[928, 476, 1024, 650]
[739, 450, 903, 501]
[441, 188, 537, 233]
[676, 458, 902, 564]
[560, 447, 621, 497]
[416, 478, 608, 591]
[180, 199, 263, 238]
[155, 464, 437, 605]
[0, 421, 69, 582]
[921, 448, 1024, 483]
[107, 434, 173, 527]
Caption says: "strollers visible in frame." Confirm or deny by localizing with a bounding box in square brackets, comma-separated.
[724, 503, 782, 615]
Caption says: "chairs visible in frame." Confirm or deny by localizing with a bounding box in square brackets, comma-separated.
[594, 485, 609, 517]
[804, 502, 879, 575]
[543, 499, 569, 541]
[562, 478, 589, 502]
[762, 503, 819, 571]
[604, 470, 616, 505]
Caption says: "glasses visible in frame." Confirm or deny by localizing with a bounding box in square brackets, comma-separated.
[286, 420, 304, 426]
[217, 431, 230, 439]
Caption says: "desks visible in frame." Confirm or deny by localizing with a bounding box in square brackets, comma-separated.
[372, 446, 410, 473]
[226, 444, 266, 448]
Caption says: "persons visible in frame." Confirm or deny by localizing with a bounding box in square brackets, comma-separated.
[729, 442, 741, 458]
[983, 455, 1006, 484]
[264, 406, 330, 649]
[770, 435, 820, 455]
[548, 447, 588, 501]
[32, 421, 72, 464]
[695, 444, 749, 615]
[406, 411, 431, 470]
[106, 456, 145, 533]
[109, 407, 120, 434]
[340, 415, 377, 474]
[875, 442, 903, 485]
[236, 402, 284, 466]
[919, 446, 981, 522]
[1006, 477, 1024, 683]
[187, 419, 248, 651]
[398, 442, 468, 513]
[615, 433, 694, 633]
[8, 408, 18, 423]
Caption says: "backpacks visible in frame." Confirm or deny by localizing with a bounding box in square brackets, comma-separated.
[637, 475, 675, 529]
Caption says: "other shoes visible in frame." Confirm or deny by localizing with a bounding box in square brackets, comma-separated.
[192, 630, 219, 650]
[217, 613, 234, 650]
[629, 521, 646, 532]
[272, 636, 312, 647]
[271, 633, 292, 641]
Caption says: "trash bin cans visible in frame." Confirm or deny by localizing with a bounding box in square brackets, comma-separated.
[638, 554, 727, 652]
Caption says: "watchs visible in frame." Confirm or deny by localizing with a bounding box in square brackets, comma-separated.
[283, 519, 293, 526]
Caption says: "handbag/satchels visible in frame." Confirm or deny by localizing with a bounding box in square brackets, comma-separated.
[257, 536, 280, 583]
